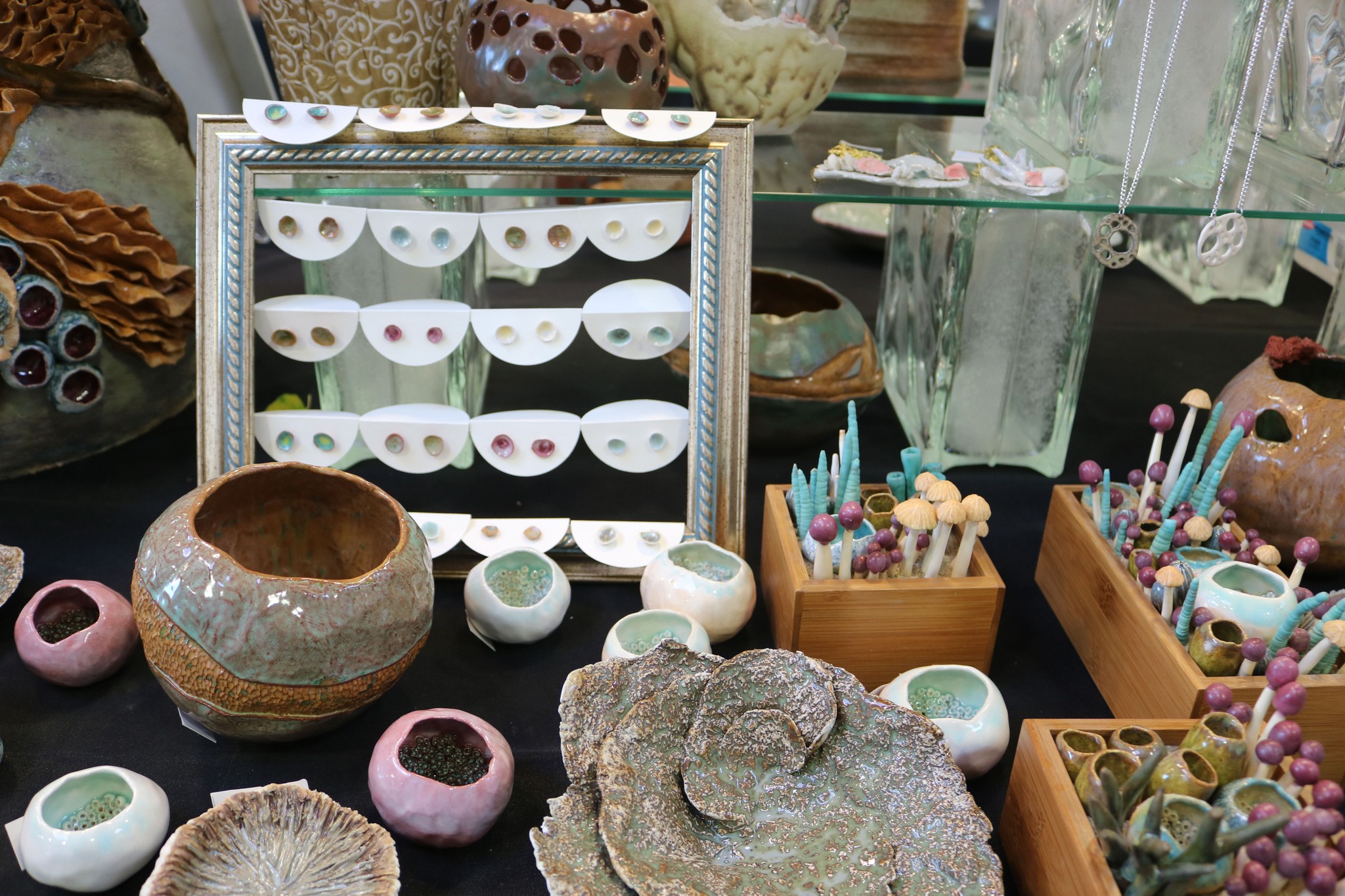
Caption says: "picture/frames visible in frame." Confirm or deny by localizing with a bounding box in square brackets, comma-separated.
[191, 113, 758, 583]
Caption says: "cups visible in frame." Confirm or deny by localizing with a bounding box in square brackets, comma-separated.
[803, 513, 877, 573]
[860, 484, 899, 530]
[1054, 710, 1303, 856]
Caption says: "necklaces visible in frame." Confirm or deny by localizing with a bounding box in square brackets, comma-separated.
[1092, 0, 1188, 269]
[1197, 0, 1297, 266]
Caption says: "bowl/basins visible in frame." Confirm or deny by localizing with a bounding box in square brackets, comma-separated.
[461, 548, 571, 642]
[874, 665, 1009, 778]
[366, 706, 516, 848]
[14, 579, 139, 687]
[128, 461, 435, 739]
[600, 611, 712, 660]
[1196, 559, 1299, 645]
[19, 764, 170, 893]
[638, 539, 758, 642]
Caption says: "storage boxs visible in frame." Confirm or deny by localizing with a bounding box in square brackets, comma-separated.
[764, 484, 1005, 686]
[1035, 484, 1345, 798]
[999, 718, 1345, 896]
